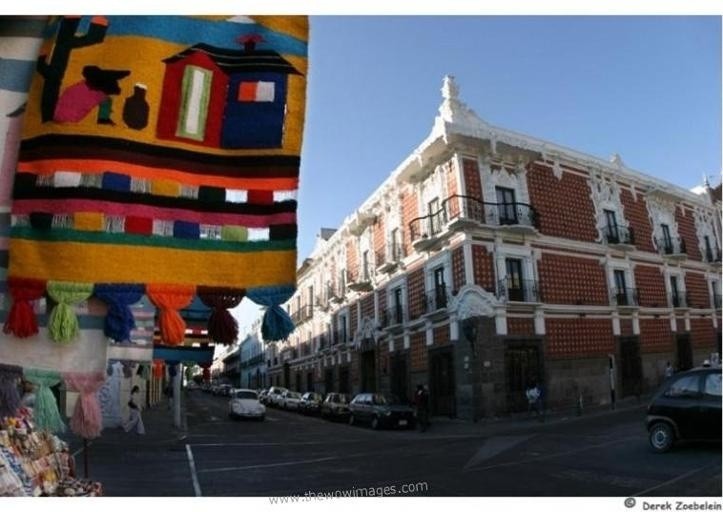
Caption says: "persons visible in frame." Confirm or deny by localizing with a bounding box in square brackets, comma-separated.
[123, 385, 147, 435]
[525, 380, 540, 418]
[411, 384, 425, 433]
[665, 361, 673, 379]
[537, 380, 546, 418]
[674, 359, 680, 375]
[424, 384, 433, 425]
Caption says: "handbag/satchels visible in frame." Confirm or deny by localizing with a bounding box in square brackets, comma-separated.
[127, 399, 139, 410]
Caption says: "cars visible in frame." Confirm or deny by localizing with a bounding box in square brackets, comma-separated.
[185, 380, 416, 432]
[646, 363, 722, 452]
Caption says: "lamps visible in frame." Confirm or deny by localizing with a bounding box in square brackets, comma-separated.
[464, 326, 477, 358]
